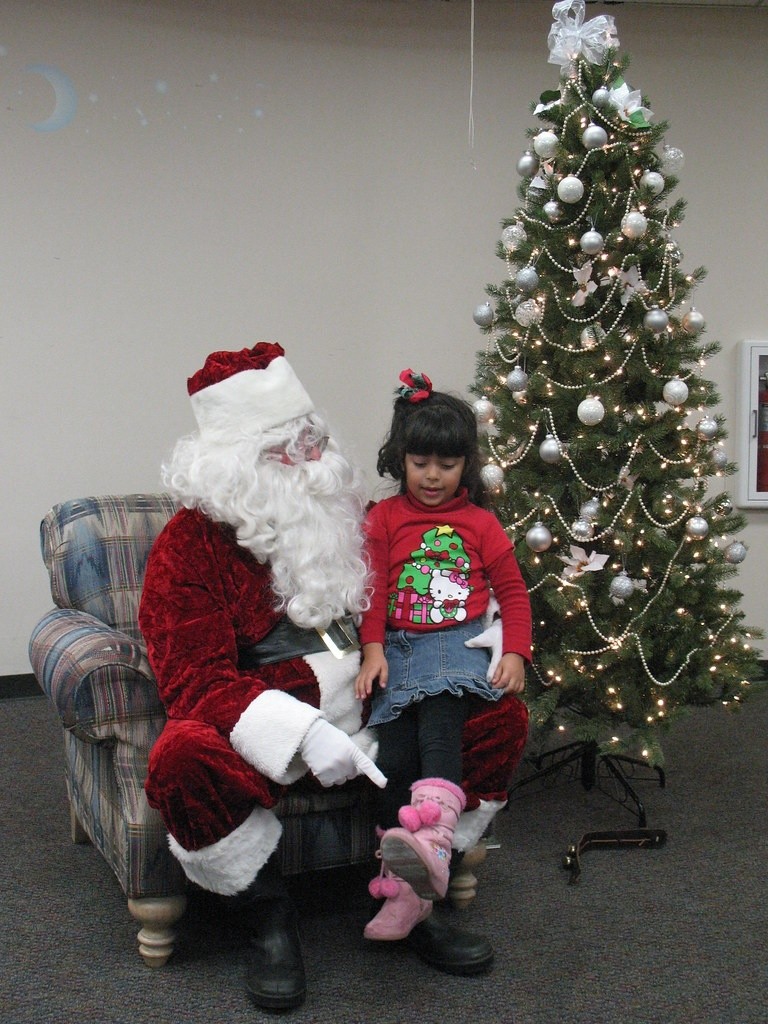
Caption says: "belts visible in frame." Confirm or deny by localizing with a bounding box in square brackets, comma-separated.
[235, 610, 359, 673]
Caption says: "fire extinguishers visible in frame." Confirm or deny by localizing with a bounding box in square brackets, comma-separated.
[757, 373, 768, 493]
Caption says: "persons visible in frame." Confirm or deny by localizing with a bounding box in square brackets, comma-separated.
[354, 368, 533, 940]
[136, 343, 530, 1009]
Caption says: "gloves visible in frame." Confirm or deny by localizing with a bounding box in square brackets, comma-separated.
[465, 617, 502, 681]
[299, 719, 388, 789]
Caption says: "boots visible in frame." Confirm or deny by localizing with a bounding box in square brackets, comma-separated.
[222, 855, 306, 1008]
[380, 776, 468, 901]
[407, 913, 494, 966]
[363, 825, 436, 940]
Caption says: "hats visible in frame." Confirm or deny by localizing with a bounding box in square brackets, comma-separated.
[186, 341, 314, 444]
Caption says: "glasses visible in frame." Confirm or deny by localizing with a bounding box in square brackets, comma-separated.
[263, 436, 330, 462]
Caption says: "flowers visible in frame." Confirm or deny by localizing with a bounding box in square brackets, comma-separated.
[610, 266, 648, 304]
[560, 544, 611, 581]
[395, 368, 433, 403]
[572, 263, 598, 304]
[608, 81, 655, 126]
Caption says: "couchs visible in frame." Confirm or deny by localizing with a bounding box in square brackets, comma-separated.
[29, 493, 528, 966]
